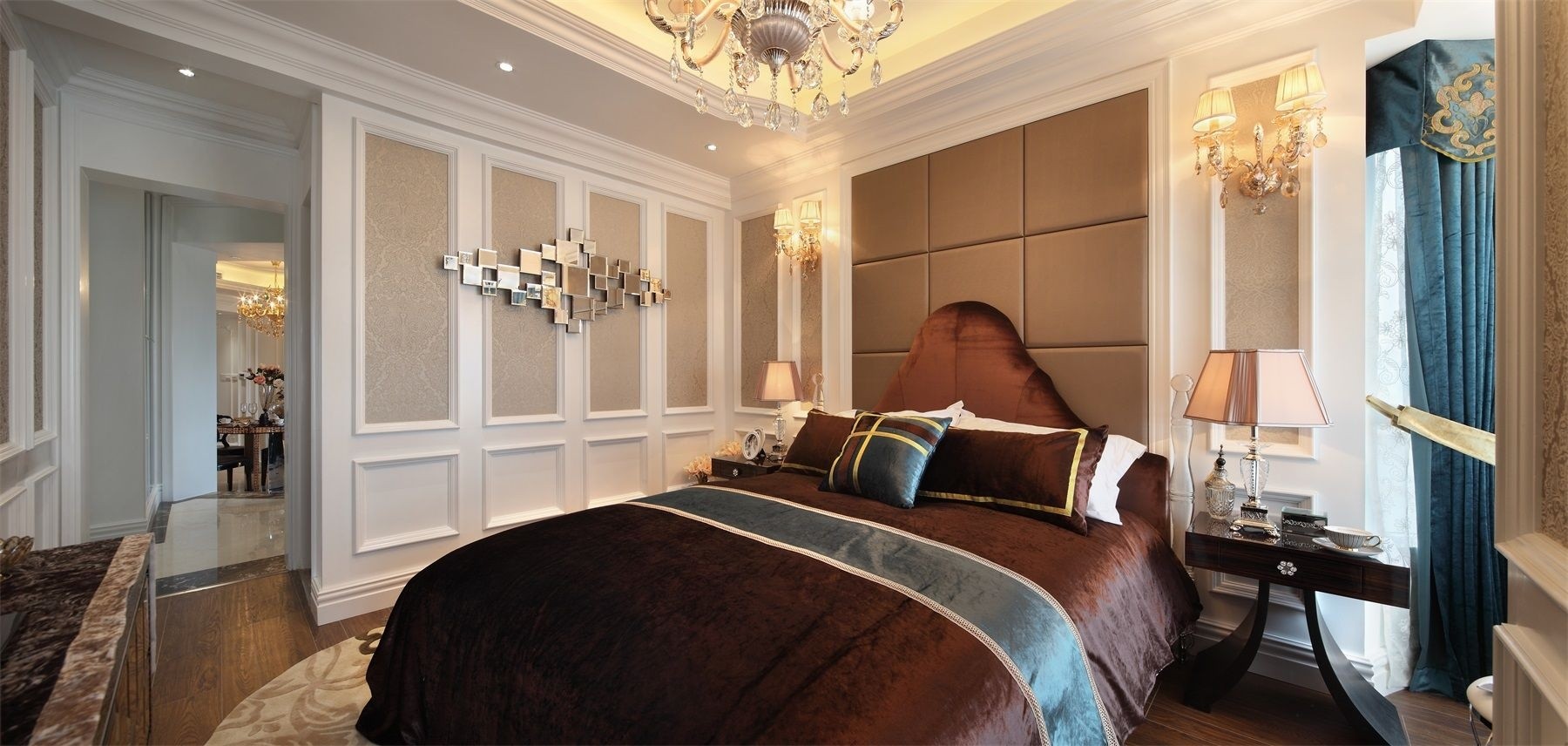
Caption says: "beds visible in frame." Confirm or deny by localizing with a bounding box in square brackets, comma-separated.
[350, 299, 1201, 746]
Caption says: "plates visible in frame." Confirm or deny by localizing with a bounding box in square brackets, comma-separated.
[1312, 537, 1384, 558]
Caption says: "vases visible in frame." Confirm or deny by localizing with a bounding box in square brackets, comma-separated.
[256, 384, 276, 423]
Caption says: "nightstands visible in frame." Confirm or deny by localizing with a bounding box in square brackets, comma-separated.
[1168, 511, 1415, 746]
[710, 450, 785, 483]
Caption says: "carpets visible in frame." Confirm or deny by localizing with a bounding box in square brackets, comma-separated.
[198, 624, 386, 745]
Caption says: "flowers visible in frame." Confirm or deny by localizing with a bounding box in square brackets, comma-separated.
[682, 454, 714, 485]
[244, 363, 284, 411]
[713, 439, 742, 458]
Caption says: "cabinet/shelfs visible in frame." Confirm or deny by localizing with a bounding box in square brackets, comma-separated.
[1, 529, 161, 746]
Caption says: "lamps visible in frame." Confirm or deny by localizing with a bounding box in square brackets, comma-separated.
[1188, 61, 1330, 212]
[1183, 347, 1332, 538]
[238, 261, 287, 340]
[772, 195, 824, 278]
[753, 358, 807, 459]
[641, 2, 905, 135]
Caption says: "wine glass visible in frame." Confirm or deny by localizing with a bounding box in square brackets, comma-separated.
[240, 403, 247, 419]
[248, 403, 257, 419]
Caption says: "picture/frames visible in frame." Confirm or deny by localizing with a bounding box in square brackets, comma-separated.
[442, 227, 673, 335]
[754, 426, 766, 453]
[743, 432, 760, 460]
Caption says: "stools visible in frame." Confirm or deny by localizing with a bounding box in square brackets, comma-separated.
[1464, 672, 1496, 744]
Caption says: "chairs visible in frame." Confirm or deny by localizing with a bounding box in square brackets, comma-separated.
[217, 414, 246, 492]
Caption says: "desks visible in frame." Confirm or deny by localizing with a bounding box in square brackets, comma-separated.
[217, 421, 285, 494]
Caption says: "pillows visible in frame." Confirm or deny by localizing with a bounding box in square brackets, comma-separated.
[782, 402, 1147, 538]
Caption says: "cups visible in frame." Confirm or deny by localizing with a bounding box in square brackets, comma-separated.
[1323, 526, 1382, 552]
[268, 404, 281, 427]
[275, 419, 284, 426]
[219, 418, 231, 424]
